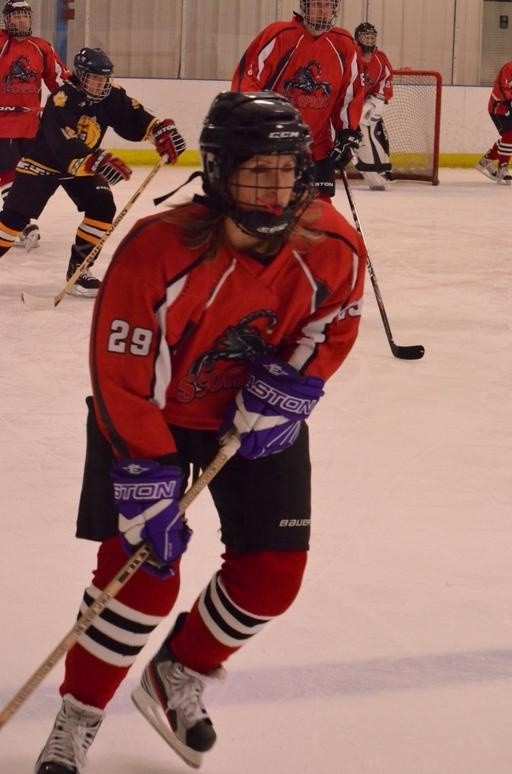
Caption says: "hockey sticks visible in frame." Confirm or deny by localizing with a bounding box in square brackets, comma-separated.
[342, 169, 424, 362]
[21, 155, 168, 309]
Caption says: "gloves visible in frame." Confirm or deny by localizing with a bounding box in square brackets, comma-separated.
[153, 118, 186, 164]
[328, 129, 362, 169]
[108, 464, 192, 579]
[85, 148, 132, 185]
[221, 358, 325, 459]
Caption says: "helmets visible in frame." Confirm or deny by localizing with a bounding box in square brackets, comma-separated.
[2, 0, 32, 36]
[73, 47, 114, 104]
[198, 89, 318, 241]
[355, 23, 377, 53]
[299, 0, 342, 37]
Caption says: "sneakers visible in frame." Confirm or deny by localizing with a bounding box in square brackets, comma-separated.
[35, 692, 105, 774]
[479, 158, 497, 177]
[66, 262, 101, 288]
[139, 643, 217, 751]
[499, 167, 512, 180]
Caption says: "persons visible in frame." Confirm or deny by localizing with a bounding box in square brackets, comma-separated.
[226, 0, 369, 201]
[0, 0, 75, 250]
[476, 57, 510, 182]
[0, 45, 185, 295]
[342, 23, 397, 169]
[38, 89, 366, 773]
